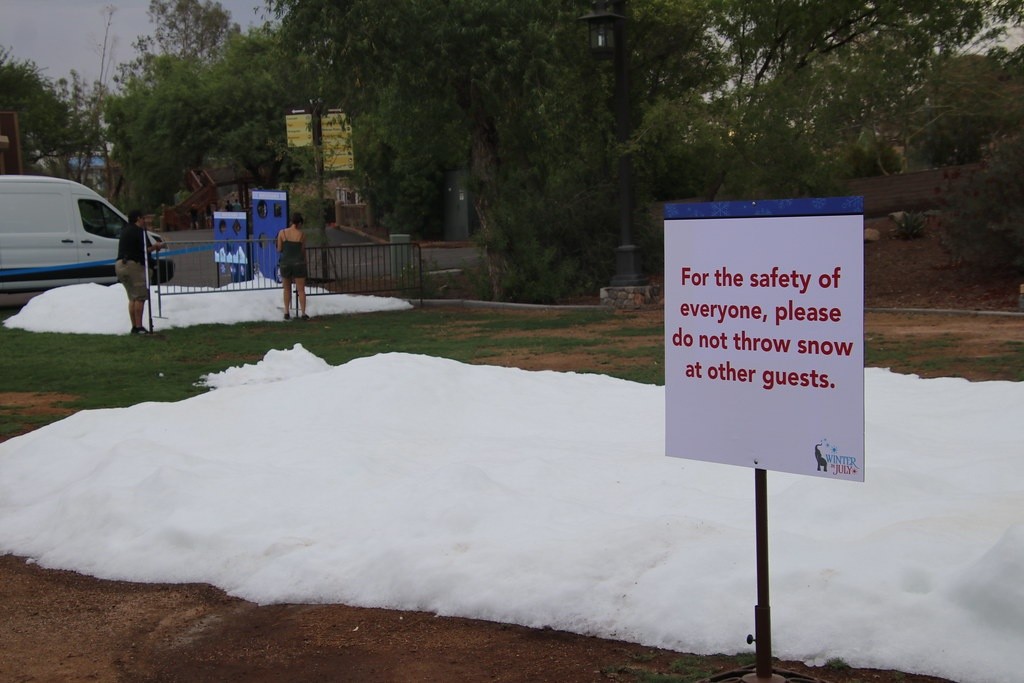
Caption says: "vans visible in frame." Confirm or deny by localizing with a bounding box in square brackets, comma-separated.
[0, 174, 175, 286]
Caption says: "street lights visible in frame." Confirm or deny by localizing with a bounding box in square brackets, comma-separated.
[575, 0, 645, 286]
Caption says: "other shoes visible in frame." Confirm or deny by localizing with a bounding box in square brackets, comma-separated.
[284, 314, 290, 319]
[131, 327, 148, 334]
[302, 315, 308, 319]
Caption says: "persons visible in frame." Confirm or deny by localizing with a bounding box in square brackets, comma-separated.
[189, 198, 242, 230]
[116, 210, 164, 335]
[275, 213, 310, 321]
